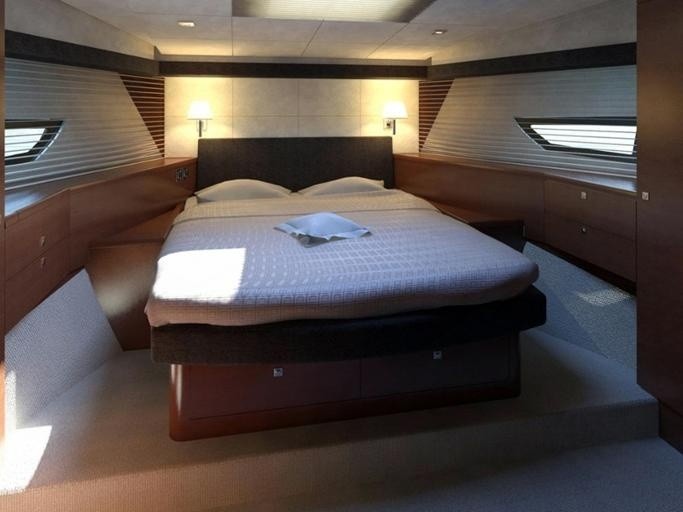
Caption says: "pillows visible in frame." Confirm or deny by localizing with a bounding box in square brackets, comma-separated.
[274, 211, 370, 241]
[194, 179, 291, 202]
[298, 176, 384, 196]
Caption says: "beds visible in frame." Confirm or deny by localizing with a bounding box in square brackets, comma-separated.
[171, 136, 522, 442]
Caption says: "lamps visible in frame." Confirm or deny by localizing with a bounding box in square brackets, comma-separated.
[187, 101, 213, 137]
[382, 101, 407, 135]
